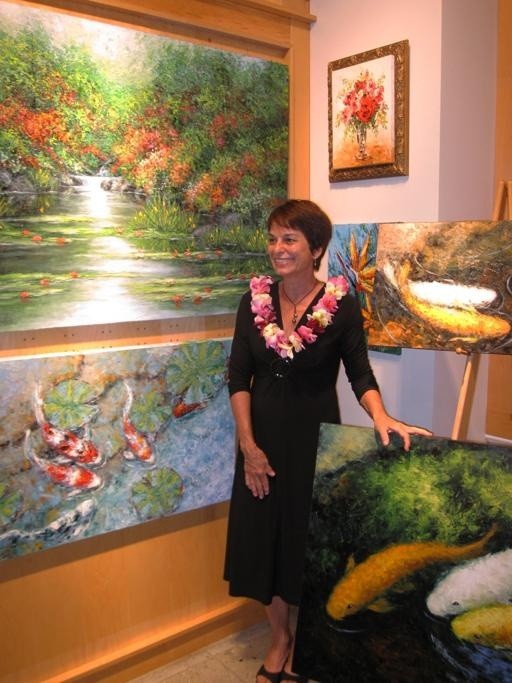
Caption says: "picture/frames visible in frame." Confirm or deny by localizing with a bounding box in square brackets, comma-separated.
[327, 39, 409, 184]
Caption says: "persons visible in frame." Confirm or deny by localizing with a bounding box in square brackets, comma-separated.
[224, 200, 433, 683]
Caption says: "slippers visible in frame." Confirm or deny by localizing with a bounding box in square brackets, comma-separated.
[256, 664, 307, 682]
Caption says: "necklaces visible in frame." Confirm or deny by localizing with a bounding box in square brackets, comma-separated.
[282, 280, 318, 322]
